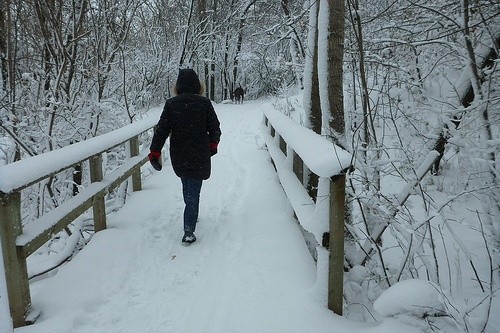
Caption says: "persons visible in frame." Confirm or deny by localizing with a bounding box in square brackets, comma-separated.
[229, 82, 244, 104]
[148, 69, 222, 246]
